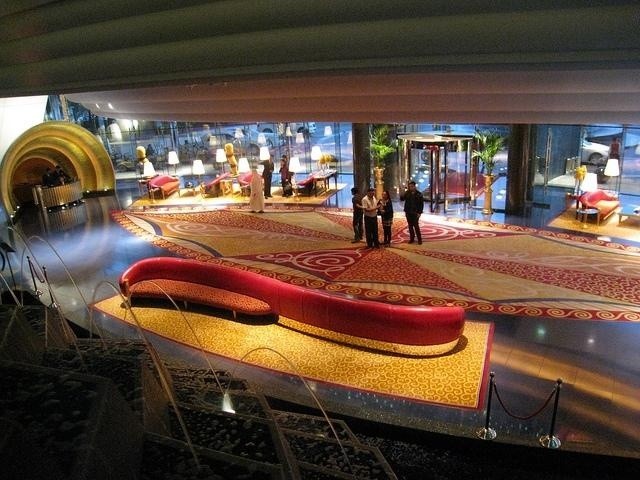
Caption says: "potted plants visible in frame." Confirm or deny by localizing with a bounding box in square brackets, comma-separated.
[471, 129, 506, 186]
[364, 124, 398, 178]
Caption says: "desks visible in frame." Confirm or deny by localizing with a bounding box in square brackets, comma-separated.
[313, 169, 338, 197]
[138, 180, 148, 197]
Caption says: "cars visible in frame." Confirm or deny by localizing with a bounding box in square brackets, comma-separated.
[581, 138, 611, 166]
[200, 121, 317, 158]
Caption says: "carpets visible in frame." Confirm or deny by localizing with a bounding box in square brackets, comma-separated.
[87, 292, 496, 413]
[545, 202, 640, 242]
[424, 172, 500, 198]
[127, 183, 347, 205]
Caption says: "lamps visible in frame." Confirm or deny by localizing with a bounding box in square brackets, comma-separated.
[581, 173, 598, 193]
[143, 146, 322, 184]
[209, 125, 352, 145]
[603, 159, 620, 191]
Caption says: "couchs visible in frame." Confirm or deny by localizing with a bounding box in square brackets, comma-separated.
[292, 172, 319, 197]
[119, 257, 465, 357]
[200, 172, 230, 198]
[149, 175, 180, 201]
[238, 173, 253, 197]
[580, 191, 620, 226]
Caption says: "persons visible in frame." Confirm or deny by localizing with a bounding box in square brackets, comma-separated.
[42, 166, 53, 184]
[257, 154, 275, 200]
[350, 188, 368, 243]
[400, 180, 424, 245]
[278, 159, 291, 198]
[145, 143, 157, 166]
[609, 135, 620, 164]
[377, 190, 393, 246]
[54, 165, 71, 180]
[281, 155, 289, 173]
[55, 176, 65, 186]
[360, 187, 382, 250]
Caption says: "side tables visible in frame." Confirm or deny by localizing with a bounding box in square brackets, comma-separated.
[577, 209, 600, 228]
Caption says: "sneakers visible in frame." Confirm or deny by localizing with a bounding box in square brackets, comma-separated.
[408, 240, 423, 245]
[351, 240, 390, 249]
